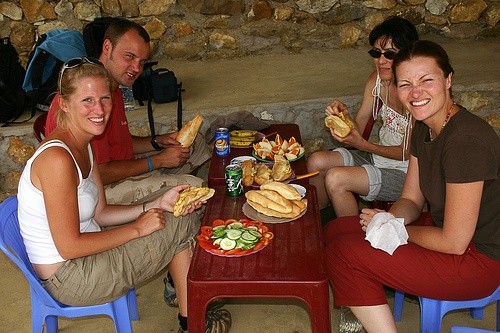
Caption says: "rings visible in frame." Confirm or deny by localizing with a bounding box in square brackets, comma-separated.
[363, 220, 366, 226]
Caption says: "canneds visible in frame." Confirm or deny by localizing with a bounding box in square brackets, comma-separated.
[225, 164, 243, 197]
[215, 128, 230, 158]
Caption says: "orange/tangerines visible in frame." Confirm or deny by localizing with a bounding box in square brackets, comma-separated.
[252, 134, 300, 160]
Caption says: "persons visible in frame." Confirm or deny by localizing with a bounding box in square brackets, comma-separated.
[321, 39, 500, 333]
[44, 20, 214, 205]
[17, 56, 232, 333]
[306, 15, 418, 217]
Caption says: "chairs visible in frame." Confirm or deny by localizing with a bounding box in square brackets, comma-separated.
[33, 113, 47, 143]
[393, 288, 500, 333]
[0, 196, 141, 333]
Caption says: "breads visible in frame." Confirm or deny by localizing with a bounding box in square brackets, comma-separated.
[239, 155, 307, 218]
[324, 112, 356, 138]
[173, 186, 215, 217]
[176, 115, 203, 147]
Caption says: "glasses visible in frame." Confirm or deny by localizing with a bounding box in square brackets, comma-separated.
[59, 56, 105, 95]
[368, 46, 399, 60]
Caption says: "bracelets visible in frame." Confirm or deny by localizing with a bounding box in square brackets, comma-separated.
[142, 201, 147, 213]
[145, 156, 153, 172]
[151, 135, 163, 151]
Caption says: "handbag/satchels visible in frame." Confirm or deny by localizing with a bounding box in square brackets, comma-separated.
[133, 60, 185, 104]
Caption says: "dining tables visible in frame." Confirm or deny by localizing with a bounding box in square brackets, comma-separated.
[208, 124, 309, 188]
[186, 185, 332, 333]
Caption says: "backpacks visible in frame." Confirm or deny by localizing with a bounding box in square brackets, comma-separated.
[0, 37, 36, 126]
[26, 34, 59, 106]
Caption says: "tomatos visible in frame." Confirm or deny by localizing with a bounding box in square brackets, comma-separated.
[195, 219, 274, 255]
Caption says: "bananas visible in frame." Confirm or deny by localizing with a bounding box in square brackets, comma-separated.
[228, 130, 257, 146]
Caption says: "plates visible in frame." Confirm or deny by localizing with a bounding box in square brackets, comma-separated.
[243, 162, 295, 187]
[231, 156, 256, 167]
[288, 183, 306, 199]
[200, 225, 269, 258]
[252, 148, 305, 162]
[229, 130, 265, 148]
[242, 200, 307, 222]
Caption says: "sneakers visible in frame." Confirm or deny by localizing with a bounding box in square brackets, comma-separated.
[163, 276, 228, 310]
[179, 309, 232, 333]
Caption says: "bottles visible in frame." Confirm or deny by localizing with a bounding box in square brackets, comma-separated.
[118, 83, 135, 111]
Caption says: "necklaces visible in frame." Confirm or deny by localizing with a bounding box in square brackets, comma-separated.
[429, 100, 456, 142]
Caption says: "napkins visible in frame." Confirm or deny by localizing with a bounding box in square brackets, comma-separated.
[364, 212, 411, 256]
